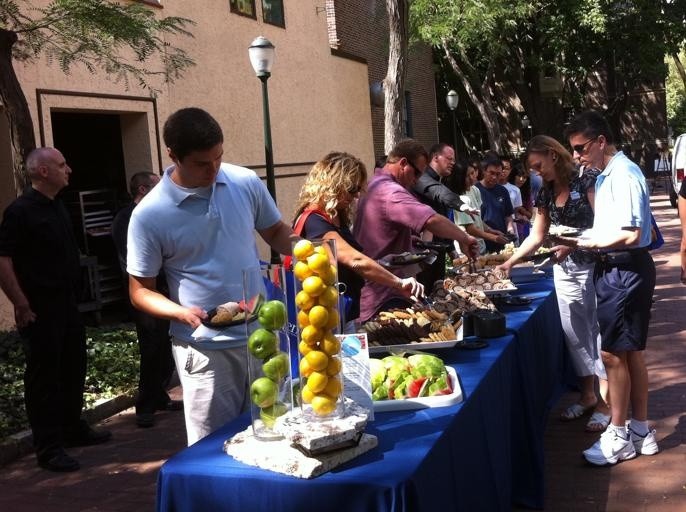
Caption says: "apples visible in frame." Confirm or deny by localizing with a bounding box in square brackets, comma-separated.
[248, 299, 290, 429]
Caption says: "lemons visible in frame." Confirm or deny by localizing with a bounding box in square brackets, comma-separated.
[293, 237, 343, 416]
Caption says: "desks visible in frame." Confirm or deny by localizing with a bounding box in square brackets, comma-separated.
[154, 267, 577, 508]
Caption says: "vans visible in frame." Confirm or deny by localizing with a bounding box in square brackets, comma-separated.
[668, 133, 685, 207]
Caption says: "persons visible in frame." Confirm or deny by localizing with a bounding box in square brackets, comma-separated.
[112, 171, 182, 429]
[127, 108, 305, 447]
[0, 147, 115, 473]
[293, 138, 533, 334]
[493, 111, 660, 463]
[675, 176, 686, 285]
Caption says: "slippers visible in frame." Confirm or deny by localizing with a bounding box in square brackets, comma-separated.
[587, 412, 611, 432]
[559, 402, 596, 421]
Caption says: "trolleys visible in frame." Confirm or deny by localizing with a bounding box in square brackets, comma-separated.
[79, 190, 128, 327]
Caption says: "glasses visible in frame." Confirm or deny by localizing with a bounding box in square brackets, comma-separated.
[406, 158, 422, 178]
[347, 186, 361, 195]
[574, 136, 599, 152]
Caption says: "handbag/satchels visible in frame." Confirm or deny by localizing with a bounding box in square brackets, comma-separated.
[649, 211, 664, 251]
[275, 263, 346, 341]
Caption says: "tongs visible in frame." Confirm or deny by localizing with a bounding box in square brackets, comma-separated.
[466, 243, 476, 275]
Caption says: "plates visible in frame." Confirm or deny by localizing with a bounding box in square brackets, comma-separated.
[200, 302, 245, 329]
[418, 242, 450, 249]
[389, 252, 428, 265]
[475, 311, 506, 339]
[514, 218, 529, 224]
[460, 340, 487, 348]
[512, 297, 531, 304]
[546, 233, 582, 238]
[522, 250, 556, 259]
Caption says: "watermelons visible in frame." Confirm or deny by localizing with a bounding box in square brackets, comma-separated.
[238, 293, 264, 316]
[366, 353, 453, 401]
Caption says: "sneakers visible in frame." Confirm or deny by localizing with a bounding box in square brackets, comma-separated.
[625, 420, 659, 455]
[582, 429, 636, 466]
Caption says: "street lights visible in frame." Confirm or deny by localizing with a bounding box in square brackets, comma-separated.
[447, 90, 460, 160]
[248, 34, 282, 264]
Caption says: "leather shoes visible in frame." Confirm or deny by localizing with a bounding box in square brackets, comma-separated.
[156, 399, 184, 411]
[35, 441, 79, 472]
[137, 413, 152, 427]
[62, 425, 112, 447]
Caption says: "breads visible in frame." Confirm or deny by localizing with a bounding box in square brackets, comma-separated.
[230, 311, 247, 322]
[210, 311, 232, 324]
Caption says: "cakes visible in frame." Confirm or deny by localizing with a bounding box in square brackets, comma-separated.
[453, 253, 512, 271]
[499, 240, 551, 257]
[357, 305, 462, 348]
[420, 277, 497, 317]
[217, 301, 238, 316]
[444, 270, 515, 293]
